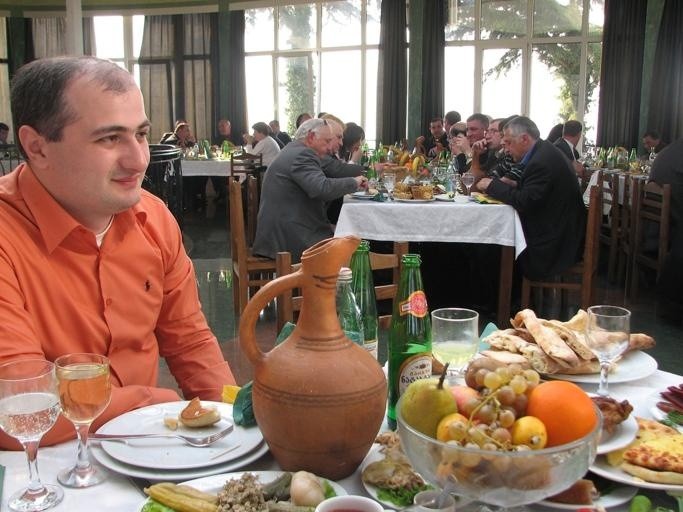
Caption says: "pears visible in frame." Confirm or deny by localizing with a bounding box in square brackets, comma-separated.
[400, 375, 457, 439]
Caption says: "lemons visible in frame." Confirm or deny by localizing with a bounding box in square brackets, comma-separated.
[435, 412, 469, 442]
[512, 416, 548, 449]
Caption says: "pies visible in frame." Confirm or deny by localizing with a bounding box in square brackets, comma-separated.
[481, 309, 656, 375]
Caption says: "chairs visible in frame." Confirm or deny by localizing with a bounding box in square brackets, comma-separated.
[276, 240, 409, 336]
[581, 165, 596, 194]
[246, 174, 272, 321]
[519, 185, 602, 318]
[629, 176, 675, 322]
[597, 168, 630, 281]
[226, 152, 263, 224]
[616, 175, 637, 301]
[229, 175, 277, 318]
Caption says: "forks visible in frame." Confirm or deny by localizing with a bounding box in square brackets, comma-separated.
[87, 424, 235, 447]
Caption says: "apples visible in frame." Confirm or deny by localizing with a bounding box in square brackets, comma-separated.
[449, 385, 481, 421]
[465, 355, 507, 393]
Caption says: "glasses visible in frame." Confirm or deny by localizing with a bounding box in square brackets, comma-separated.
[484, 129, 501, 137]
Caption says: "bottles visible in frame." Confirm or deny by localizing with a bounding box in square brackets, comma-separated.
[599, 144, 656, 168]
[335, 239, 429, 432]
[203, 140, 209, 154]
[223, 140, 228, 157]
[361, 141, 459, 179]
[184, 143, 199, 158]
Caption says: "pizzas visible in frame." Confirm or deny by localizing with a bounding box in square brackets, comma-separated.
[606, 416, 683, 485]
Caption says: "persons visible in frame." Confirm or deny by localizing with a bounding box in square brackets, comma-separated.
[213, 118, 239, 146]
[160, 119, 187, 144]
[416, 109, 589, 283]
[252, 112, 371, 264]
[641, 132, 683, 262]
[0, 121, 10, 145]
[243, 119, 292, 181]
[164, 123, 196, 147]
[0, 55, 236, 450]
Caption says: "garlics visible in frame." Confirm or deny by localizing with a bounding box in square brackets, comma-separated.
[290, 471, 325, 506]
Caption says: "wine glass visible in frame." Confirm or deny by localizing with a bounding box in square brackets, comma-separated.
[431, 307, 479, 374]
[0, 353, 111, 512]
[586, 305, 632, 395]
[383, 166, 475, 203]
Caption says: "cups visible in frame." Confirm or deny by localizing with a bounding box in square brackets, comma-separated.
[237, 146, 243, 156]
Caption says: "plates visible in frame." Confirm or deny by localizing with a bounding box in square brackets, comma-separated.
[354, 191, 372, 199]
[90, 400, 269, 480]
[141, 351, 683, 511]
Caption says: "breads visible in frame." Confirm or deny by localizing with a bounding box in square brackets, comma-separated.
[143, 482, 220, 512]
[178, 397, 223, 429]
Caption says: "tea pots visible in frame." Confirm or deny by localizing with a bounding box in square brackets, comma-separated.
[240, 237, 386, 484]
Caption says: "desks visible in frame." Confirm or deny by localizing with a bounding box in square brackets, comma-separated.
[589, 168, 651, 205]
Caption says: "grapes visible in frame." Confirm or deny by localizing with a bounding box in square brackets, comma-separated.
[443, 363, 541, 468]
